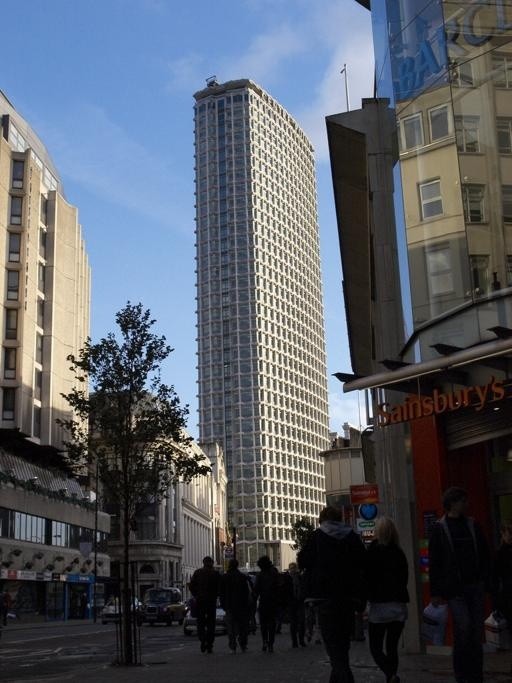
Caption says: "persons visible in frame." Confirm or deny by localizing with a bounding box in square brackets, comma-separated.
[301, 506, 366, 683]
[189, 556, 322, 654]
[428, 487, 498, 683]
[357, 516, 410, 683]
[489, 518, 512, 640]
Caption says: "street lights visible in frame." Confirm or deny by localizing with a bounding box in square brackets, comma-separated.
[94, 457, 108, 623]
[228, 526, 236, 559]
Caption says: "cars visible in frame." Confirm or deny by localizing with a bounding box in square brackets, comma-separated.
[137, 586, 185, 627]
[100, 595, 142, 624]
[184, 598, 192, 612]
[183, 596, 227, 636]
